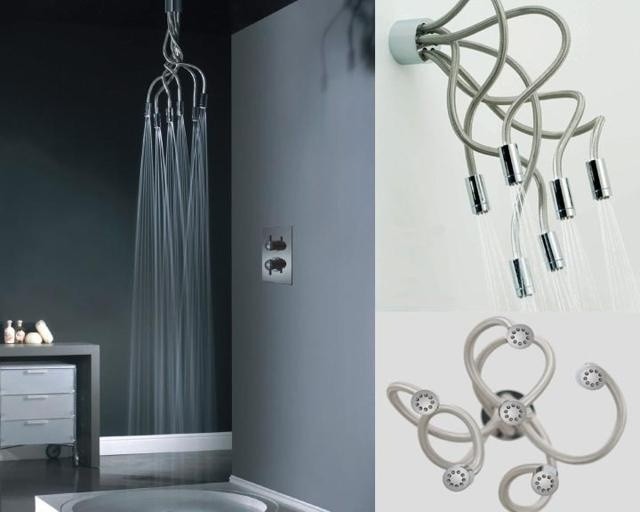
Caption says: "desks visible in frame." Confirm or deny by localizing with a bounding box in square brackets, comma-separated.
[0, 340, 100, 469]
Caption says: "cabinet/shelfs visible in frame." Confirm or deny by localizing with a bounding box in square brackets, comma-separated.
[0, 362, 83, 466]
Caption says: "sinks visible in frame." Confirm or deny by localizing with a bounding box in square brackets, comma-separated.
[33, 482, 318, 512]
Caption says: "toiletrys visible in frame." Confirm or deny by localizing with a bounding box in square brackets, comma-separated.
[4, 320, 15, 344]
[15, 320, 25, 343]
